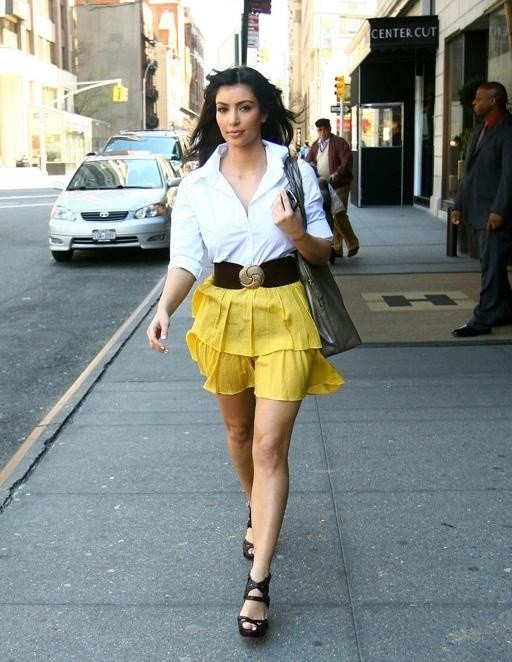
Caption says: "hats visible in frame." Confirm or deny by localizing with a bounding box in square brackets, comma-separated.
[314, 119, 330, 127]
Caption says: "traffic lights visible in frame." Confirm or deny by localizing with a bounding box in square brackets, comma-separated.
[334, 75, 346, 98]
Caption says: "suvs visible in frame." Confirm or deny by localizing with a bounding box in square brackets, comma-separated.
[103, 130, 200, 177]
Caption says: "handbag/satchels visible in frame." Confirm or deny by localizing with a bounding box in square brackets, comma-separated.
[297, 252, 361, 357]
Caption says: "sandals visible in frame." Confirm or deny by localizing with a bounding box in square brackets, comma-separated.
[243, 505, 256, 559]
[237, 572, 272, 637]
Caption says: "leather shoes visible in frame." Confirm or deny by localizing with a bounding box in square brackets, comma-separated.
[453, 323, 491, 337]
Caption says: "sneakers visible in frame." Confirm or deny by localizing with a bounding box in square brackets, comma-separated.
[347, 247, 359, 257]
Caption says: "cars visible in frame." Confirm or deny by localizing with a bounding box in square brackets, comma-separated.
[48, 156, 183, 262]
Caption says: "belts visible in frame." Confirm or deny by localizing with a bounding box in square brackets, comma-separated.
[214, 257, 300, 289]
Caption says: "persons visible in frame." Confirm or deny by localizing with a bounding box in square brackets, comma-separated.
[304, 118, 363, 262]
[449, 81, 510, 339]
[304, 141, 311, 158]
[148, 67, 344, 640]
